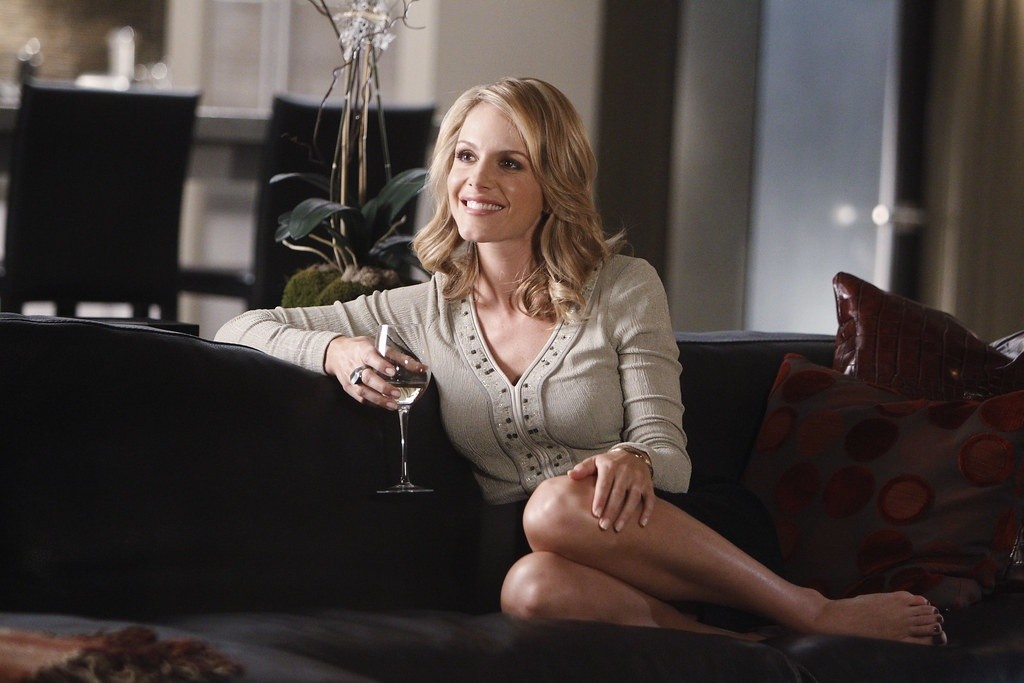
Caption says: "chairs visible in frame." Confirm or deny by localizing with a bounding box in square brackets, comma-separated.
[0, 76, 203, 320]
[248, 94, 437, 312]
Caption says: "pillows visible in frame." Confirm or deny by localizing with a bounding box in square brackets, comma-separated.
[740, 272, 1024, 617]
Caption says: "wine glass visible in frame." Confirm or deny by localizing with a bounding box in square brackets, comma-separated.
[376, 324, 433, 495]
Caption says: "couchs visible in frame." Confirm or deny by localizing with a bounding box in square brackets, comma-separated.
[0, 320, 1024, 683]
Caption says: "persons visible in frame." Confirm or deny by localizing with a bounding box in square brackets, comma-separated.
[214, 77, 946, 647]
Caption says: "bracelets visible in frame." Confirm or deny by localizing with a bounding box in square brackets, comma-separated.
[610, 447, 654, 478]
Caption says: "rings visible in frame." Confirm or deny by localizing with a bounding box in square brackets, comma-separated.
[350, 366, 373, 385]
